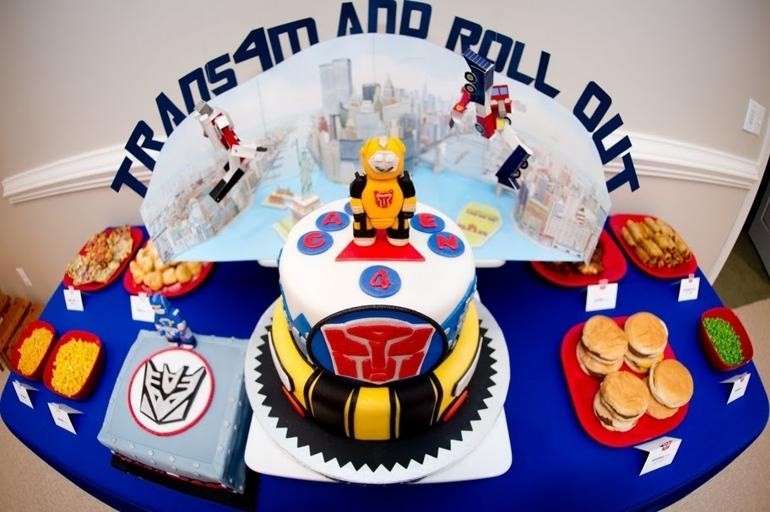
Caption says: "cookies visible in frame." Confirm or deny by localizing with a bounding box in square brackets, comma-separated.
[621, 216, 689, 268]
[66, 228, 202, 292]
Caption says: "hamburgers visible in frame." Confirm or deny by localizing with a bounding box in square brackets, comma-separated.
[576, 311, 693, 432]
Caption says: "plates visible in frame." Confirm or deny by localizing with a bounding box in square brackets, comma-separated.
[609, 213, 700, 279]
[58, 226, 146, 294]
[43, 328, 103, 401]
[560, 313, 689, 449]
[122, 234, 211, 298]
[6, 318, 57, 382]
[530, 229, 627, 289]
[702, 306, 755, 374]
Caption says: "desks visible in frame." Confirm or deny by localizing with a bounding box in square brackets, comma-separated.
[0, 221, 770, 511]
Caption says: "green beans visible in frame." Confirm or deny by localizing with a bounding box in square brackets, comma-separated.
[704, 318, 745, 365]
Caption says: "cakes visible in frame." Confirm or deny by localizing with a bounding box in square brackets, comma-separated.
[96, 294, 249, 499]
[268, 136, 483, 443]
